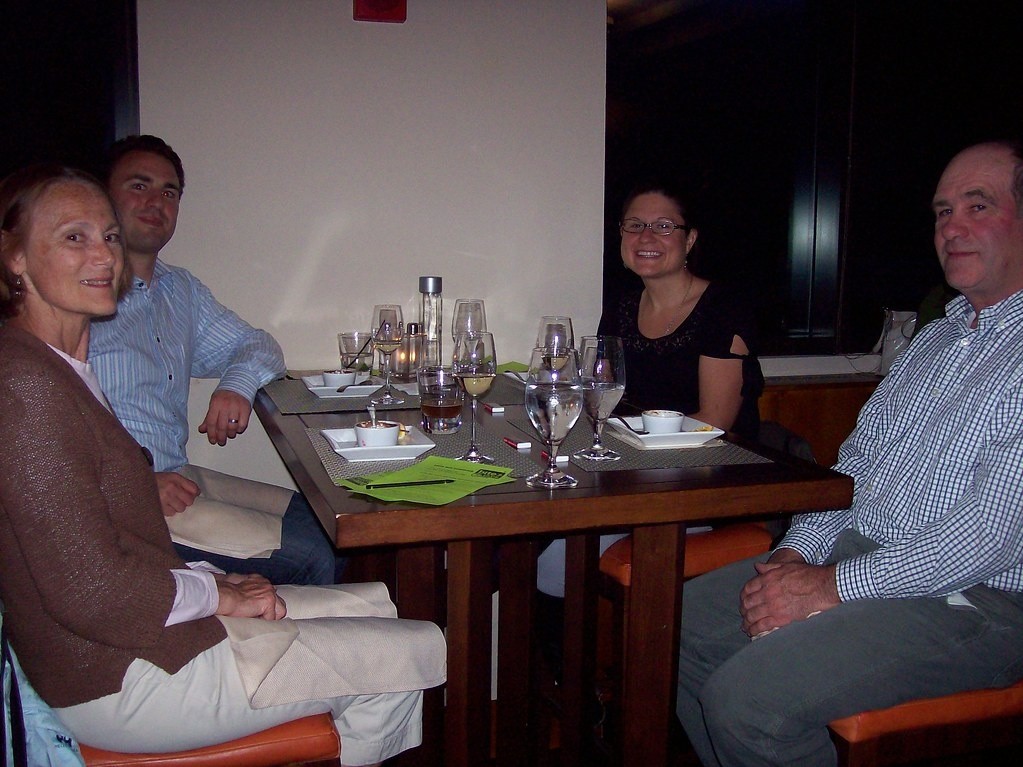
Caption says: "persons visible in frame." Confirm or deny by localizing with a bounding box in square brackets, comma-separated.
[674, 142, 1022, 767]
[92, 133, 352, 587]
[528, 181, 766, 724]
[0, 160, 426, 767]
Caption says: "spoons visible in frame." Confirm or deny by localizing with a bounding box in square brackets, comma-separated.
[336, 380, 372, 393]
[610, 413, 650, 435]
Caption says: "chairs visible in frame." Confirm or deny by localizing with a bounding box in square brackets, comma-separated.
[1, 626, 342, 767]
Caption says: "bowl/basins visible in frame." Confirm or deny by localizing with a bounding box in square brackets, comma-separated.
[354, 420, 400, 447]
[642, 409, 685, 434]
[322, 369, 356, 387]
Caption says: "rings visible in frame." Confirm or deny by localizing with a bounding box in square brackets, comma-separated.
[227, 419, 239, 425]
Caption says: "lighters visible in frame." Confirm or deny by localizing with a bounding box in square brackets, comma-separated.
[504, 435, 531, 449]
[483, 402, 505, 413]
[540, 447, 570, 462]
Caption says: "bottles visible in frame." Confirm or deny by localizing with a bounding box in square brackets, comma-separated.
[417, 275, 443, 366]
[406, 324, 425, 380]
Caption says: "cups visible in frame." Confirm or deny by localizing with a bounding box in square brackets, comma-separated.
[337, 332, 375, 375]
[418, 366, 463, 435]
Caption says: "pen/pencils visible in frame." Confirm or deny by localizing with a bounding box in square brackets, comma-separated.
[365, 480, 455, 489]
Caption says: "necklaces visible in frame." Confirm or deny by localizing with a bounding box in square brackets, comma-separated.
[640, 274, 694, 338]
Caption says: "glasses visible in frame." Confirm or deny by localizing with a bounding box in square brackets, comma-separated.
[618, 217, 690, 236]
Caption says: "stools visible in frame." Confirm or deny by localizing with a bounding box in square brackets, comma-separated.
[827, 685, 1023, 767]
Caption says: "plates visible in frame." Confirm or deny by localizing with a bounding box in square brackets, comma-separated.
[606, 414, 725, 446]
[300, 375, 386, 398]
[505, 371, 594, 392]
[319, 425, 436, 464]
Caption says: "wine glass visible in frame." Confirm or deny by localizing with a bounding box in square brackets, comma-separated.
[451, 299, 488, 395]
[537, 316, 574, 390]
[573, 335, 625, 463]
[453, 330, 494, 465]
[370, 304, 404, 405]
[525, 347, 583, 489]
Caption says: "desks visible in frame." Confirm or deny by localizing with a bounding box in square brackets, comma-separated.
[253, 368, 854, 767]
